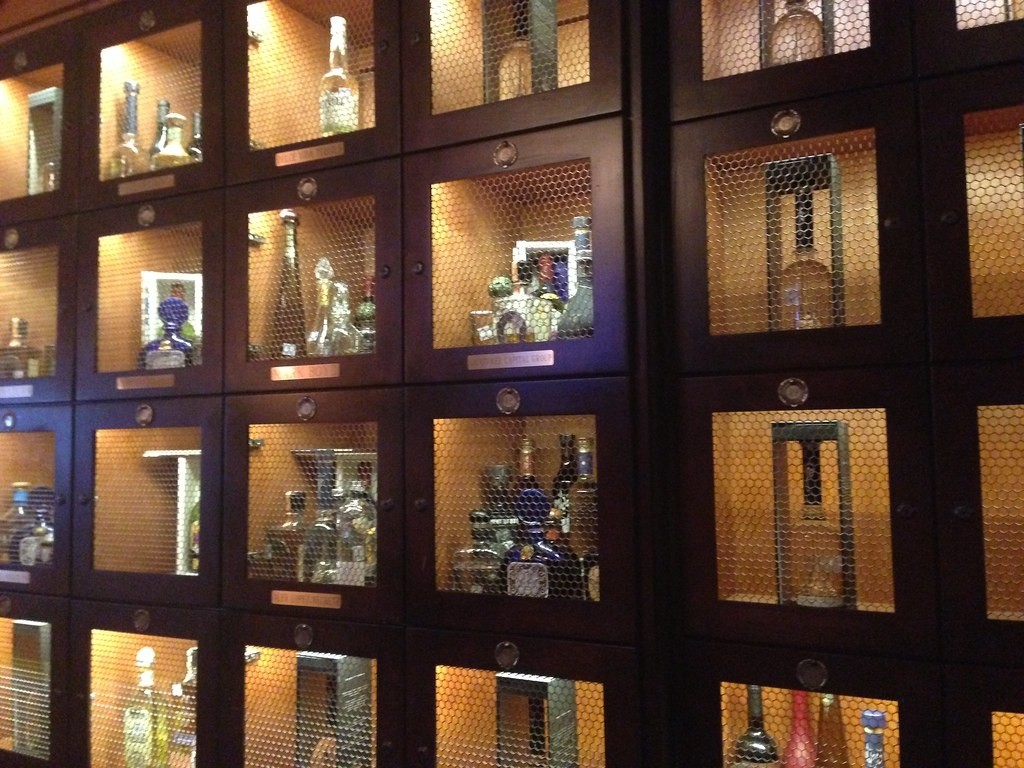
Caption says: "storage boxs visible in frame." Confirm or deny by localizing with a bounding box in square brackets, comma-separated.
[761, 146, 850, 329]
[10, 617, 50, 760]
[758, 0, 835, 69]
[494, 671, 577, 768]
[481, 0, 560, 103]
[769, 412, 861, 612]
[292, 652, 372, 768]
[25, 87, 62, 196]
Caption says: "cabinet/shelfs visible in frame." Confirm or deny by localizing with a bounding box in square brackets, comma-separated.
[0, 0, 1024, 768]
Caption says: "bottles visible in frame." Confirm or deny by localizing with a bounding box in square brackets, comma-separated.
[123, 647, 167, 768]
[41, 147, 59, 192]
[498, 0, 533, 101]
[780, 186, 832, 332]
[138, 296, 194, 371]
[186, 111, 203, 163]
[188, 500, 200, 573]
[861, 709, 886, 768]
[8, 485, 56, 571]
[0, 317, 42, 380]
[816, 693, 852, 768]
[734, 685, 780, 768]
[488, 216, 594, 345]
[306, 674, 336, 768]
[769, 0, 823, 68]
[454, 432, 599, 599]
[110, 80, 148, 180]
[271, 210, 374, 361]
[25, 686, 41, 752]
[248, 450, 375, 586]
[318, 16, 359, 138]
[0, 482, 35, 562]
[152, 113, 192, 170]
[518, 697, 547, 768]
[790, 439, 841, 606]
[169, 646, 198, 755]
[150, 99, 171, 165]
[782, 690, 816, 768]
